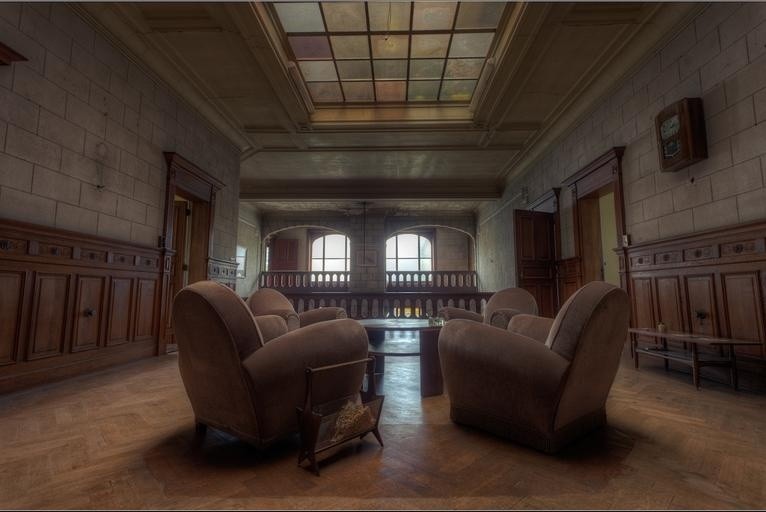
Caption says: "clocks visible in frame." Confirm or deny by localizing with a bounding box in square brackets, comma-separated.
[654, 97, 708, 183]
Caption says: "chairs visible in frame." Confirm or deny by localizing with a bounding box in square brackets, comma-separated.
[172, 280, 369, 465]
[438, 280, 630, 454]
[437, 287, 539, 331]
[246, 288, 347, 333]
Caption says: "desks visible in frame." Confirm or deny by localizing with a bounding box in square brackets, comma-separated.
[627, 327, 764, 391]
